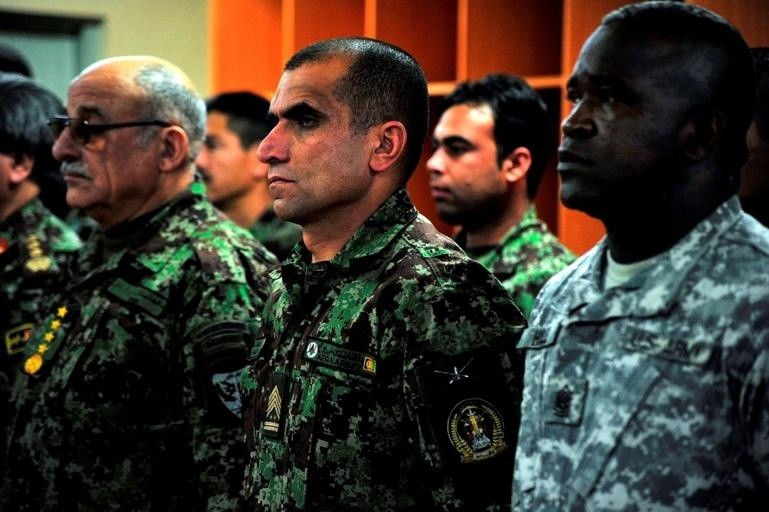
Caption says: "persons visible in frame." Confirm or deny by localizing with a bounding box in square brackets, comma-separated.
[233, 31, 533, 509]
[430, 69, 585, 322]
[200, 85, 304, 257]
[0, 50, 283, 510]
[505, 1, 767, 509]
[0, 72, 88, 442]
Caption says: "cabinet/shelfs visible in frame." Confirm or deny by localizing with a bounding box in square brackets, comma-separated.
[204, 0, 769, 257]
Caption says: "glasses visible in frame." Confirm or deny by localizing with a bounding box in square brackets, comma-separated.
[46, 115, 171, 146]
